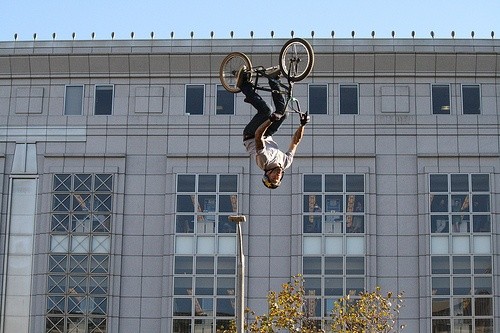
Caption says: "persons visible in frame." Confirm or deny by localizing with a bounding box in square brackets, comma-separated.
[235, 65, 309, 190]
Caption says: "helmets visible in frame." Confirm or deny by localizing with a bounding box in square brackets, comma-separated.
[262, 179, 284, 189]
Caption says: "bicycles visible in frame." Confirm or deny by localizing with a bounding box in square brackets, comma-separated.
[220, 38, 314, 123]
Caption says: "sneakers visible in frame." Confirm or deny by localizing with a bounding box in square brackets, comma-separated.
[234, 65, 247, 89]
[268, 69, 284, 82]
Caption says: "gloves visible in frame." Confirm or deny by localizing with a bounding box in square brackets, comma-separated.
[269, 111, 284, 123]
[300, 111, 310, 127]
